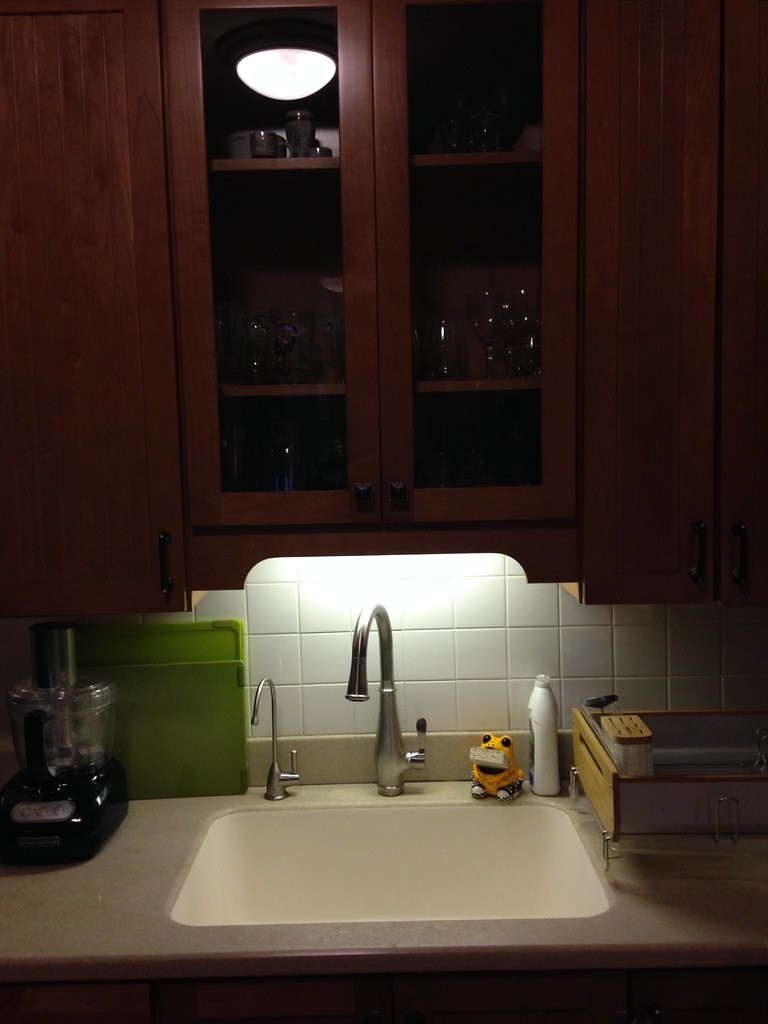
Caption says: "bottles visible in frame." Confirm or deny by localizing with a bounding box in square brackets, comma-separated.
[528, 675, 561, 796]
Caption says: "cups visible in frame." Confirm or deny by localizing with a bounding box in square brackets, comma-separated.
[221, 425, 247, 491]
[316, 434, 347, 490]
[249, 112, 332, 158]
[270, 444, 295, 490]
[463, 105, 501, 151]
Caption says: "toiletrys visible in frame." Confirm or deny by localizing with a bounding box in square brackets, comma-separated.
[527, 673, 562, 797]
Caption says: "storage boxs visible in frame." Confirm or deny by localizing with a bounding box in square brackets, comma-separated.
[571, 708, 768, 843]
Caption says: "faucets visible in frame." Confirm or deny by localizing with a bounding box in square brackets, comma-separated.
[344, 598, 428, 796]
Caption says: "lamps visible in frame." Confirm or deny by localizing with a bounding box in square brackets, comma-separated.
[212, 19, 338, 101]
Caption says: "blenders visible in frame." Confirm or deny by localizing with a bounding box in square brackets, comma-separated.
[0, 621, 128, 864]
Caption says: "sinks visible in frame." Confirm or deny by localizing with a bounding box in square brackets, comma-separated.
[165, 780, 613, 930]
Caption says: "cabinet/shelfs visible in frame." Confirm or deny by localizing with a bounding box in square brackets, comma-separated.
[0, 965, 768, 1024]
[0, 1, 768, 616]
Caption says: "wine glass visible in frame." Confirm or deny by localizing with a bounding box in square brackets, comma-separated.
[428, 318, 457, 379]
[467, 288, 540, 378]
[217, 304, 311, 385]
